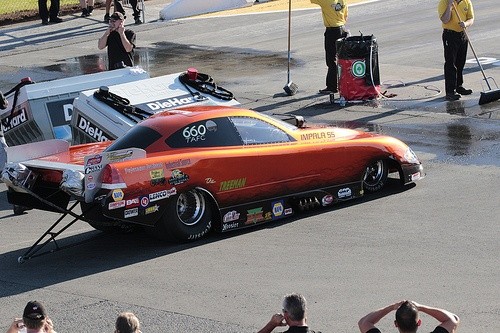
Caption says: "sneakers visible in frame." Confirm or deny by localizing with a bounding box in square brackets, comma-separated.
[318, 87, 337, 94]
[446, 87, 472, 99]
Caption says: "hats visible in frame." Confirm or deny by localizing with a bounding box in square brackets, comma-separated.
[108, 11, 124, 20]
[23, 300, 47, 320]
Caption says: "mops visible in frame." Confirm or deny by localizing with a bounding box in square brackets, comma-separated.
[451, 0, 500, 106]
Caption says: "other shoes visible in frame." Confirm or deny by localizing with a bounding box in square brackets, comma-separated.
[104, 15, 110, 22]
[81, 6, 94, 17]
[135, 17, 142, 24]
[42, 18, 63, 24]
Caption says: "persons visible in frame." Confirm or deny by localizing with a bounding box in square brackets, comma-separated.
[5, 300, 58, 333]
[114, 0, 142, 24]
[257, 292, 322, 333]
[104, 0, 118, 22]
[114, 312, 143, 333]
[38, 0, 63, 24]
[310, 0, 349, 93]
[97, 12, 136, 70]
[438, 0, 474, 99]
[357, 299, 460, 333]
[80, 0, 94, 17]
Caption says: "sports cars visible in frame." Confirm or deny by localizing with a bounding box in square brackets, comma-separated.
[3, 107, 426, 244]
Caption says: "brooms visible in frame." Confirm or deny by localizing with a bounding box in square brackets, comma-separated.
[282, 0, 300, 96]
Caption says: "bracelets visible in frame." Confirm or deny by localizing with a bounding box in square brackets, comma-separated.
[104, 33, 109, 36]
